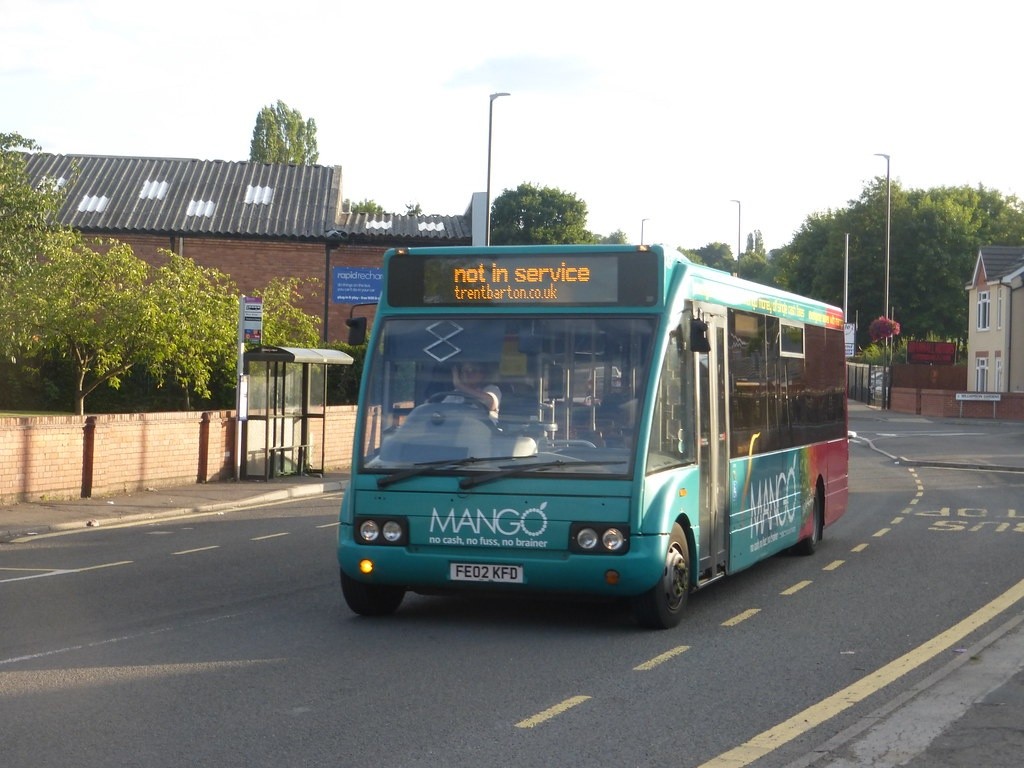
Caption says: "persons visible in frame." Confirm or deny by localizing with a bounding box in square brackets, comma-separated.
[442, 350, 502, 427]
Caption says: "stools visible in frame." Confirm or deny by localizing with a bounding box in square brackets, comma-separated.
[260, 445, 310, 479]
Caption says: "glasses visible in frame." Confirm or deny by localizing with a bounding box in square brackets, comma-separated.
[459, 367, 480, 374]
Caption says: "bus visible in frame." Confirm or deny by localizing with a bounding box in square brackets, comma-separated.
[337, 246, 850, 628]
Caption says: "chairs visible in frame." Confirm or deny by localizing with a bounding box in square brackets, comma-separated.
[568, 391, 628, 448]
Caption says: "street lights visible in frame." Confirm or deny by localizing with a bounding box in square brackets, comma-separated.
[641, 218, 648, 244]
[486, 92, 512, 246]
[874, 153, 892, 410]
[732, 199, 741, 278]
